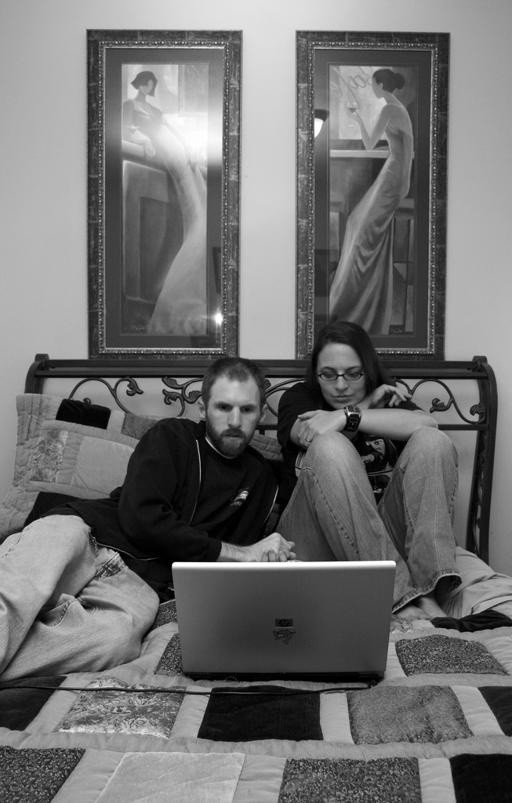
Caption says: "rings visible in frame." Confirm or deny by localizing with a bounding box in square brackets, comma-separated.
[305, 439, 312, 442]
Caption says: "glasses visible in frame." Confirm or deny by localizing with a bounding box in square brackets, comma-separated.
[316, 371, 364, 381]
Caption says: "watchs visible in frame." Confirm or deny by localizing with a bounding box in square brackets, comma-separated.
[343, 404, 362, 432]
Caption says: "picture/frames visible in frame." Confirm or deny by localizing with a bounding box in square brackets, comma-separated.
[295, 29, 450, 363]
[86, 29, 242, 362]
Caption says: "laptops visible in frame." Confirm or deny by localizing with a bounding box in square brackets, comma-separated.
[171, 560, 396, 683]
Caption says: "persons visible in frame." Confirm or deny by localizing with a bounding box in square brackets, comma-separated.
[0, 359, 293, 683]
[265, 322, 462, 623]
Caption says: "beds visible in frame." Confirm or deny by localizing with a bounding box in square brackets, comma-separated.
[1, 355, 512, 803]
